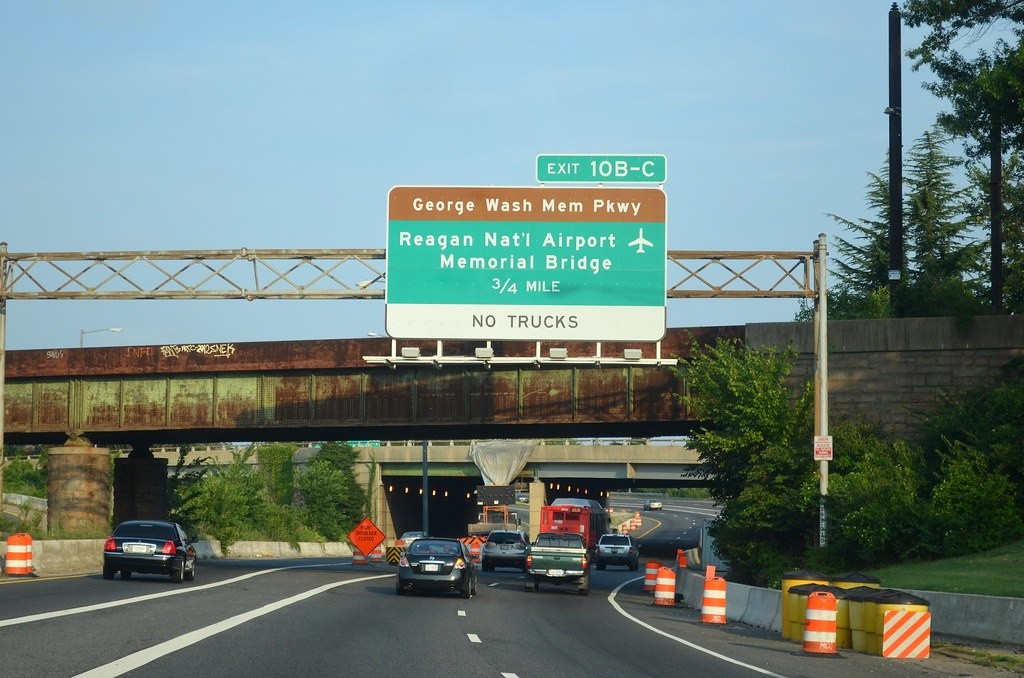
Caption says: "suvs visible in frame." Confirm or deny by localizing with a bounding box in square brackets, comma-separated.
[481, 528, 529, 576]
[595, 531, 640, 573]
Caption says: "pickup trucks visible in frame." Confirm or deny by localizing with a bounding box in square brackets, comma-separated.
[525, 531, 591, 595]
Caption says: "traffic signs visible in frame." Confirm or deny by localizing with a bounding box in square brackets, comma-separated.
[385, 184, 666, 344]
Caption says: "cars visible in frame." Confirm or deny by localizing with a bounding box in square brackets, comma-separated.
[643, 497, 664, 511]
[98, 518, 194, 584]
[400, 530, 429, 546]
[397, 538, 478, 598]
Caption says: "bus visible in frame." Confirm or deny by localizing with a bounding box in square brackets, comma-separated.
[538, 497, 611, 562]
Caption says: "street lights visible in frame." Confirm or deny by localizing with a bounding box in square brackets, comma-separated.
[77, 326, 123, 346]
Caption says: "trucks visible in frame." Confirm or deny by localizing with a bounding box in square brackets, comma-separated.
[466, 506, 521, 534]
